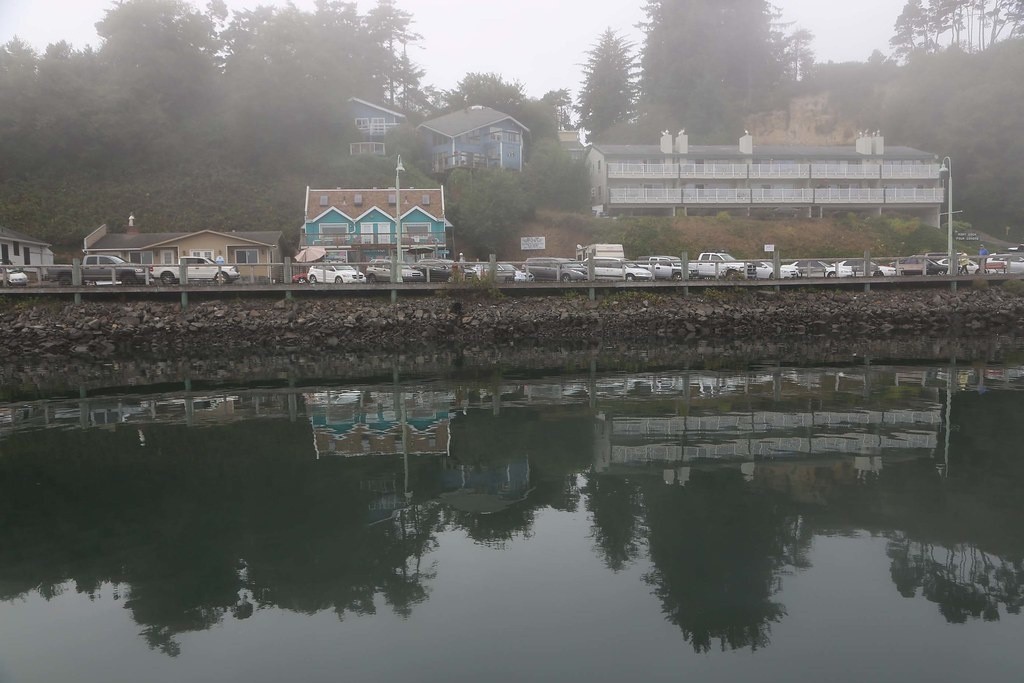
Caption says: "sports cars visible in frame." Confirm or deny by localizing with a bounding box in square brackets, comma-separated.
[755, 262, 798, 279]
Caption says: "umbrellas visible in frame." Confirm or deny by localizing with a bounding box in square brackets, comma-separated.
[293, 246, 326, 262]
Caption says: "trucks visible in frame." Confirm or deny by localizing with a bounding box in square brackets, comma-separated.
[633, 256, 700, 281]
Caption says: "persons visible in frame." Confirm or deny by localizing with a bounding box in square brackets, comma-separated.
[959, 250, 970, 274]
[978, 244, 989, 269]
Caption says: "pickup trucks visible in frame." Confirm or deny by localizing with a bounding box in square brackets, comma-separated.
[687, 253, 757, 279]
[149, 256, 241, 285]
[53, 405, 144, 429]
[47, 255, 155, 285]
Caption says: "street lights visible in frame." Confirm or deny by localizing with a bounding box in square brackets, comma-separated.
[939, 156, 953, 274]
[395, 154, 405, 283]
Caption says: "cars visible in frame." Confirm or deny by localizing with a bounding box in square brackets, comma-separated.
[521, 258, 588, 283]
[365, 259, 424, 284]
[888, 256, 948, 275]
[818, 259, 896, 278]
[472, 264, 535, 283]
[410, 259, 477, 283]
[581, 257, 652, 282]
[307, 262, 366, 284]
[780, 262, 799, 277]
[291, 269, 309, 283]
[936, 258, 998, 276]
[0, 262, 28, 287]
